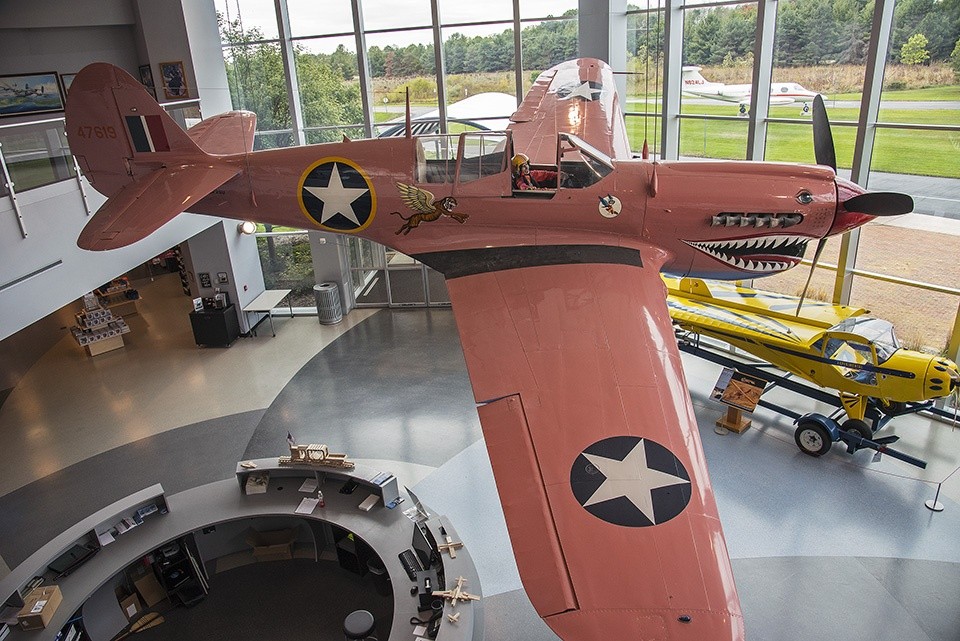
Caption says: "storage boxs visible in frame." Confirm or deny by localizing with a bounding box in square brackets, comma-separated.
[242, 524, 301, 561]
[115, 582, 142, 619]
[16, 584, 63, 631]
[128, 560, 165, 608]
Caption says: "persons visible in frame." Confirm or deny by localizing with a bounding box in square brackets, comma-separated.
[511, 153, 568, 191]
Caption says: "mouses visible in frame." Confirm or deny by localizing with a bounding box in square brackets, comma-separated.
[411, 586, 418, 595]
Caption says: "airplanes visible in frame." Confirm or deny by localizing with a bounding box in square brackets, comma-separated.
[65, 59, 915, 641]
[682, 67, 827, 114]
[657, 272, 960, 469]
[432, 575, 480, 607]
[437, 536, 464, 559]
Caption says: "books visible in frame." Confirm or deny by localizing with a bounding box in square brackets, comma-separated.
[98, 517, 135, 547]
[0, 606, 23, 641]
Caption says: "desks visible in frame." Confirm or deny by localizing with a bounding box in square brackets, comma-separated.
[242, 289, 294, 337]
[189, 304, 241, 348]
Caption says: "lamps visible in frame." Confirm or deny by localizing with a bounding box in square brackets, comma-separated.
[237, 221, 257, 235]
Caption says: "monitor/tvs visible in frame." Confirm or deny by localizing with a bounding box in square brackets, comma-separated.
[412, 521, 433, 570]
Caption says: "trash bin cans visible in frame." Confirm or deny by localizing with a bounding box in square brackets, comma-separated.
[312, 282, 342, 325]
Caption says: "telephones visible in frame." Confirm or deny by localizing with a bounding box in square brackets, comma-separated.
[426, 608, 444, 637]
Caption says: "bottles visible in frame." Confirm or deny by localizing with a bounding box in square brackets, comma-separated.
[318, 491, 325, 507]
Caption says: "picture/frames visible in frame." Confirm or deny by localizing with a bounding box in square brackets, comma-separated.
[139, 64, 158, 102]
[60, 72, 77, 97]
[0, 70, 66, 118]
[159, 61, 189, 99]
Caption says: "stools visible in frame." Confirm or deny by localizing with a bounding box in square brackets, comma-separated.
[343, 610, 378, 641]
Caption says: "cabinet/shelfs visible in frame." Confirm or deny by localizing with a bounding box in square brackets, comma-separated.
[70, 287, 143, 357]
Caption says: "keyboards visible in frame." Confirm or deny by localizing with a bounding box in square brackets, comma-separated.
[399, 548, 423, 580]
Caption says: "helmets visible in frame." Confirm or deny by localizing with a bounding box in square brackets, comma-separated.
[511, 153, 529, 173]
